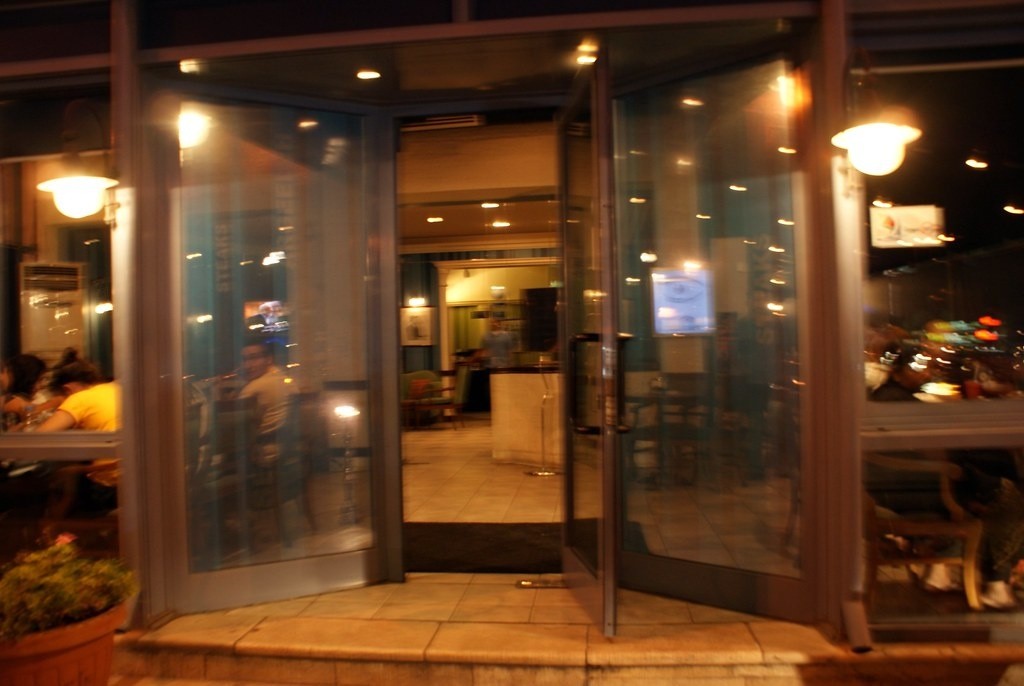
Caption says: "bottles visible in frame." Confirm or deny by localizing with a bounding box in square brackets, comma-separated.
[22, 406, 37, 436]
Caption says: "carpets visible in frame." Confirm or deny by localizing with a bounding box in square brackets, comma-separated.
[401, 521, 650, 575]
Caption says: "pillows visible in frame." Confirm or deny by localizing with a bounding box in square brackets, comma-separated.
[408, 378, 432, 399]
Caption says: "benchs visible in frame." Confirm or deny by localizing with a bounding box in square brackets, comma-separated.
[401, 371, 444, 430]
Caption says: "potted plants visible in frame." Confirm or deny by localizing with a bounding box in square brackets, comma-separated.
[0, 542, 139, 686]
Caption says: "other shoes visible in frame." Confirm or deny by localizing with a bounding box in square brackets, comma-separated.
[925, 560, 964, 590]
[977, 580, 1016, 606]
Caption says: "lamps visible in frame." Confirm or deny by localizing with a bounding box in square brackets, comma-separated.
[333, 401, 360, 525]
[830, 49, 922, 176]
[37, 101, 132, 219]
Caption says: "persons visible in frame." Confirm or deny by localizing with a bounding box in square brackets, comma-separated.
[185, 378, 210, 482]
[726, 284, 784, 485]
[38, 346, 121, 522]
[197, 337, 302, 568]
[472, 318, 514, 368]
[862, 350, 1024, 608]
[0, 353, 48, 513]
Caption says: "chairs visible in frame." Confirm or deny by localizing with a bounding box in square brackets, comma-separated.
[413, 364, 471, 430]
[215, 393, 320, 549]
[862, 449, 986, 612]
